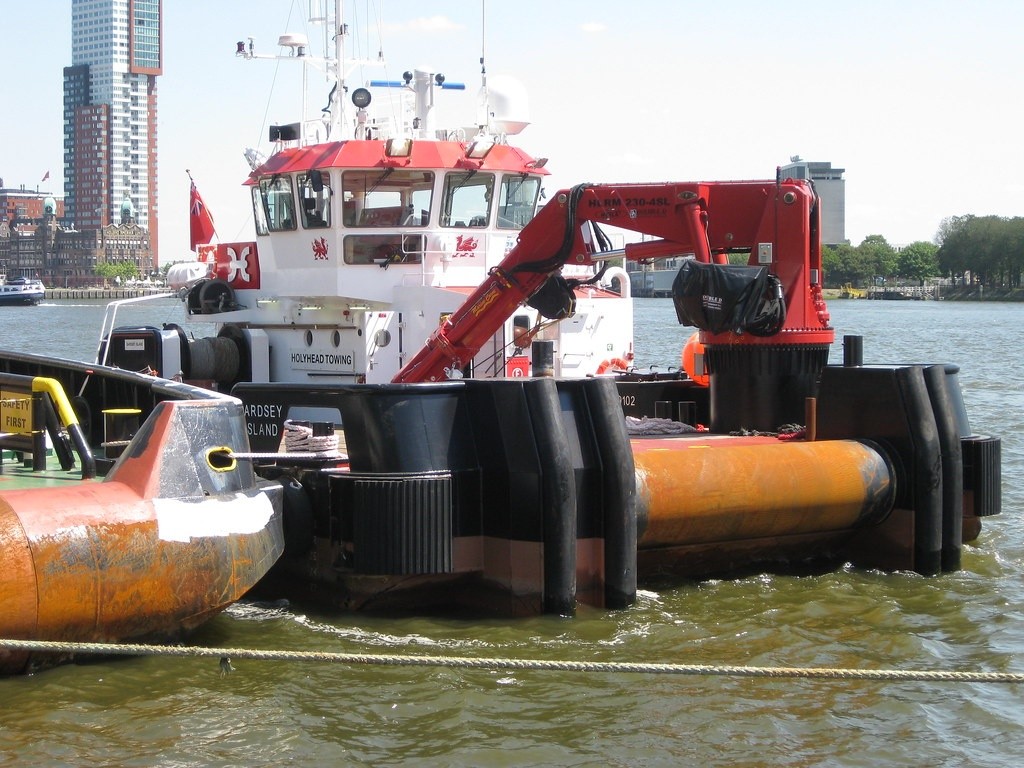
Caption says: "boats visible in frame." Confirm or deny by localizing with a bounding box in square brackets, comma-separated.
[0, 274, 46, 306]
[2, 72, 1003, 663]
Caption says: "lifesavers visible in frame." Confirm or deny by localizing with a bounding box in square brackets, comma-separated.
[596, 358, 628, 375]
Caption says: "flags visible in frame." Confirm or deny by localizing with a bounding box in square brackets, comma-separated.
[41, 170, 49, 182]
[187, 180, 215, 253]
